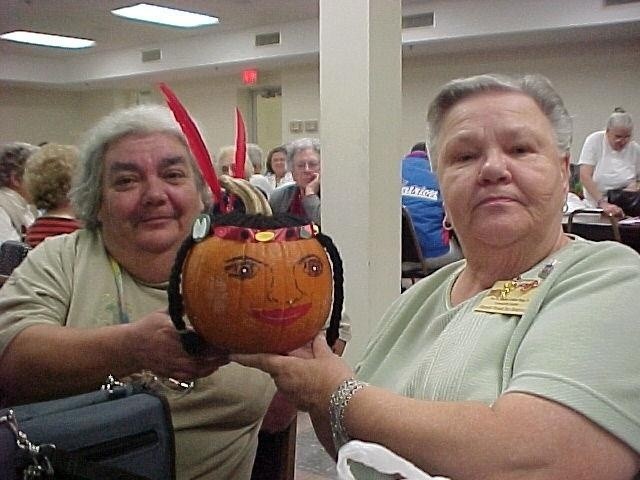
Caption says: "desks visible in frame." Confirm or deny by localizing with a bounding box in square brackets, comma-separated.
[560, 210, 639, 252]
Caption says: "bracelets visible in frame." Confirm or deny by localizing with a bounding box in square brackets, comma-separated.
[327, 377, 369, 449]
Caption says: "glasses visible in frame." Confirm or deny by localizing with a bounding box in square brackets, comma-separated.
[614, 136, 632, 144]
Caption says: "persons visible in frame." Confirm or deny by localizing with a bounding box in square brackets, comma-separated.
[229, 73, 640, 480]
[0, 104, 279, 480]
[0, 142, 84, 250]
[213, 138, 322, 226]
[577, 108, 640, 219]
[402, 141, 463, 271]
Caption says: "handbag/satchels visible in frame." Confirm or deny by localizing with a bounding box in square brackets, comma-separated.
[0, 378, 176, 480]
[607, 189, 640, 217]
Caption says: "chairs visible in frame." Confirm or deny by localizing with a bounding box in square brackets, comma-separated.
[402, 206, 439, 292]
